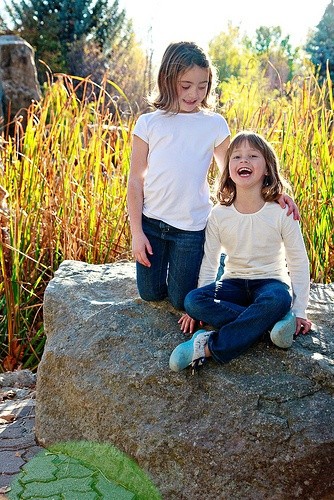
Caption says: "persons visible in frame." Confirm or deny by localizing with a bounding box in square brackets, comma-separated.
[127, 40, 302, 310]
[168, 134, 312, 375]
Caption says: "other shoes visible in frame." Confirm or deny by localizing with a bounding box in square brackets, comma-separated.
[169, 329, 215, 375]
[270, 311, 297, 348]
